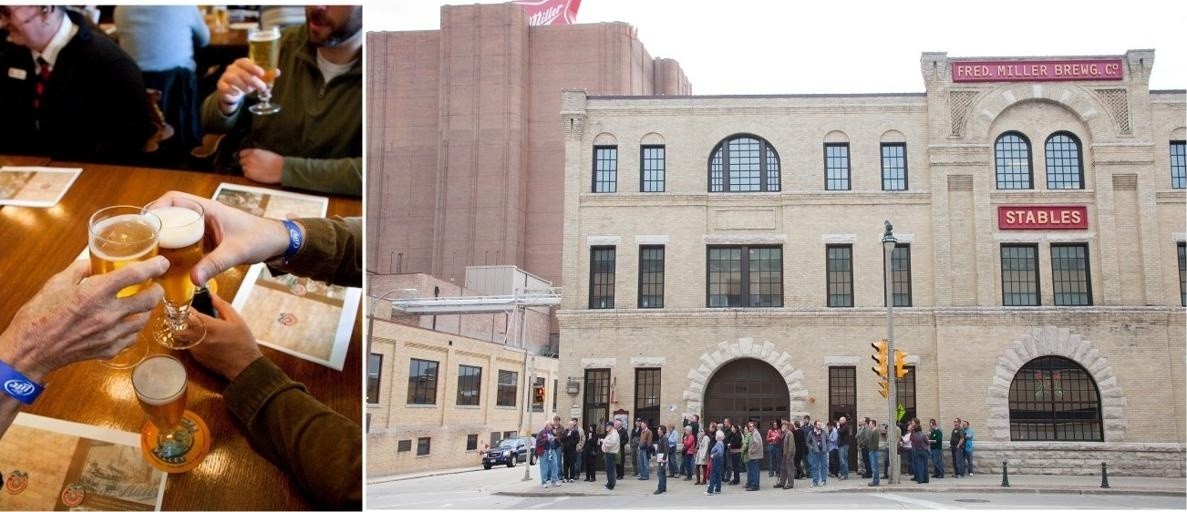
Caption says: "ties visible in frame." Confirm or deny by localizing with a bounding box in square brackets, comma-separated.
[34, 56, 50, 118]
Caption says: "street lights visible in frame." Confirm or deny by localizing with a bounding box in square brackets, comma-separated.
[366, 287, 420, 393]
[880, 219, 901, 486]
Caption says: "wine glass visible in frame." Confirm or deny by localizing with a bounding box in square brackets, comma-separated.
[129, 352, 194, 460]
[246, 22, 283, 117]
[88, 202, 164, 371]
[142, 196, 207, 352]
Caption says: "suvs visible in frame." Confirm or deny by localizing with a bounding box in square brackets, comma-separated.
[480, 436, 539, 468]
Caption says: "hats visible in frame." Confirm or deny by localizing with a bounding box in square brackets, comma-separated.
[604, 421, 614, 426]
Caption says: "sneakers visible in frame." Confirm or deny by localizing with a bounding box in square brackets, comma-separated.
[774, 484, 792, 489]
[747, 488, 756, 491]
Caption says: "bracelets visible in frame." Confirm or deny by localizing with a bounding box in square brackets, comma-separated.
[281, 220, 301, 261]
[0, 359, 45, 406]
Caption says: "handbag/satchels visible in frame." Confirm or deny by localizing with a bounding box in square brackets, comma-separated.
[590, 449, 598, 457]
[657, 453, 669, 463]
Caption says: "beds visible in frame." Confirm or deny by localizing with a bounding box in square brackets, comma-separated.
[0, 159, 362, 510]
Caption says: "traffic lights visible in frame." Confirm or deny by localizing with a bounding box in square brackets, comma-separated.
[535, 388, 545, 404]
[896, 351, 909, 378]
[877, 381, 887, 400]
[871, 340, 888, 378]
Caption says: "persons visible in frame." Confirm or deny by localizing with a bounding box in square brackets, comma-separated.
[141, 189, 361, 510]
[0, 5, 148, 167]
[527, 414, 973, 495]
[200, 6, 360, 200]
[112, 5, 210, 70]
[0, 256, 170, 439]
